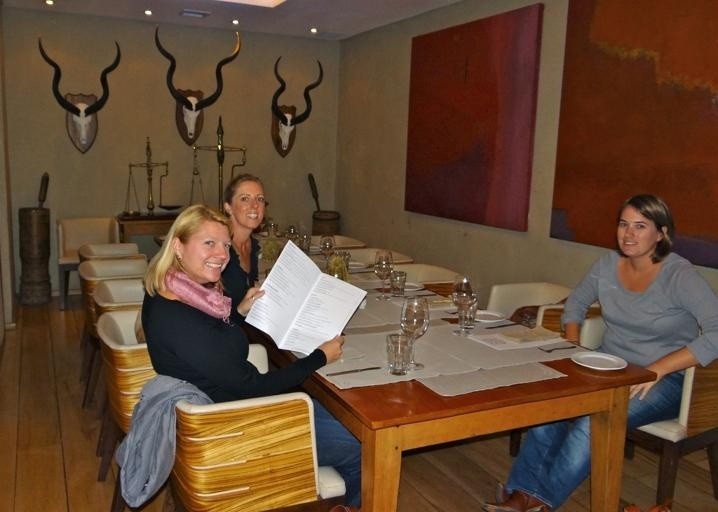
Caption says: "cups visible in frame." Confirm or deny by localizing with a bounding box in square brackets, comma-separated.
[338, 250, 351, 277]
[464, 295, 478, 328]
[386, 334, 414, 375]
[390, 271, 406, 297]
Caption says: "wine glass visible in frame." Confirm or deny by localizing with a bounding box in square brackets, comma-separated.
[453, 275, 472, 335]
[375, 251, 393, 301]
[400, 297, 430, 370]
[320, 234, 336, 273]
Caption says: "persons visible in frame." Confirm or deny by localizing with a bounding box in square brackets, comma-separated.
[219, 174, 267, 306]
[140, 202, 361, 512]
[483, 192, 718, 511]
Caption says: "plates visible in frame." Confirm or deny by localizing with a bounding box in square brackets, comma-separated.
[349, 261, 369, 270]
[404, 282, 426, 291]
[475, 310, 506, 322]
[571, 351, 629, 371]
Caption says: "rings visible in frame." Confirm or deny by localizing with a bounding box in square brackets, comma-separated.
[252, 295, 255, 301]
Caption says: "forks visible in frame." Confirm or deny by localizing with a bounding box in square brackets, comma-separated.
[536, 344, 576, 353]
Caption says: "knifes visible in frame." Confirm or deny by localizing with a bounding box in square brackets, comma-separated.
[326, 360, 382, 377]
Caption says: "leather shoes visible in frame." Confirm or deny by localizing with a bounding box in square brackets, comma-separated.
[492, 481, 511, 503]
[480, 490, 551, 512]
